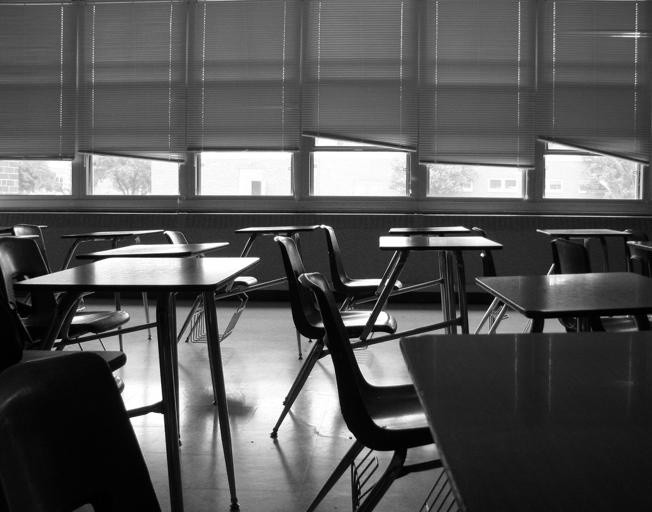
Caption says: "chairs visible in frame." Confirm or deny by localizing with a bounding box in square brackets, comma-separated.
[473, 223, 630, 335]
[0, 235, 130, 352]
[521, 238, 635, 333]
[270, 236, 504, 439]
[300, 271, 444, 512]
[163, 230, 256, 345]
[627, 239, 651, 276]
[1, 352, 164, 512]
[11, 224, 107, 352]
[319, 224, 472, 336]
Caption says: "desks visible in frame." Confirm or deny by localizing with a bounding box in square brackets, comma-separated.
[14, 255, 261, 511]
[75, 241, 230, 347]
[475, 273, 651, 333]
[397, 332, 651, 512]
[60, 229, 164, 352]
[235, 225, 321, 360]
